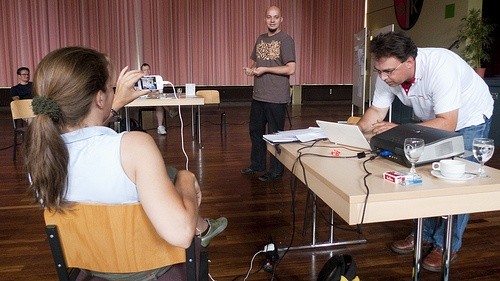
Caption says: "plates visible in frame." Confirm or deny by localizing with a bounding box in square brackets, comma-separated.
[431, 170, 477, 181]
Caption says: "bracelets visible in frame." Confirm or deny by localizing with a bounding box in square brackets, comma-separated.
[110, 108, 118, 115]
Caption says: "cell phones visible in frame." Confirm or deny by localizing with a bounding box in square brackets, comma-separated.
[138, 75, 164, 92]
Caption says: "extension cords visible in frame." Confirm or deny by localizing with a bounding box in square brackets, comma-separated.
[263, 247, 278, 271]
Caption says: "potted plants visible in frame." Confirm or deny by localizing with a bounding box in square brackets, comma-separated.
[455, 7, 497, 78]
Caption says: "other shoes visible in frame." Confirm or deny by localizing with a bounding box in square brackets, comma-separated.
[258, 169, 282, 181]
[240, 166, 265, 174]
[199, 216, 227, 248]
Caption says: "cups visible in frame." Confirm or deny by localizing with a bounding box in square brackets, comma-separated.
[177, 88, 183, 98]
[432, 160, 466, 177]
[151, 92, 158, 98]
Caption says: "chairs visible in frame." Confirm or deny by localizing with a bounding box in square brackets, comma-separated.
[10, 98, 34, 163]
[194, 90, 227, 139]
[139, 106, 167, 134]
[43, 201, 208, 281]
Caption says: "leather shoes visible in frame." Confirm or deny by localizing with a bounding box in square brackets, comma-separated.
[391, 232, 434, 254]
[422, 248, 459, 271]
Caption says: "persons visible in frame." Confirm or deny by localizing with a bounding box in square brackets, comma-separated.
[21, 45, 229, 281]
[11, 67, 37, 126]
[137, 62, 178, 135]
[354, 30, 496, 271]
[240, 6, 297, 181]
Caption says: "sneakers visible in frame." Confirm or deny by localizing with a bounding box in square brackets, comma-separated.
[157, 126, 166, 134]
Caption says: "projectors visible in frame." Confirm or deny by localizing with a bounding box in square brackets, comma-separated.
[369, 123, 465, 168]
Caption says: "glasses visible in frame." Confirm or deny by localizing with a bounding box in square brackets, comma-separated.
[372, 63, 402, 75]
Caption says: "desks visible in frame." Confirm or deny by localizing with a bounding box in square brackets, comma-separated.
[124, 93, 205, 150]
[265, 141, 500, 281]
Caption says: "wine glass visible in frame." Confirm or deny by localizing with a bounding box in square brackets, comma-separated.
[472, 138, 495, 179]
[404, 138, 424, 179]
